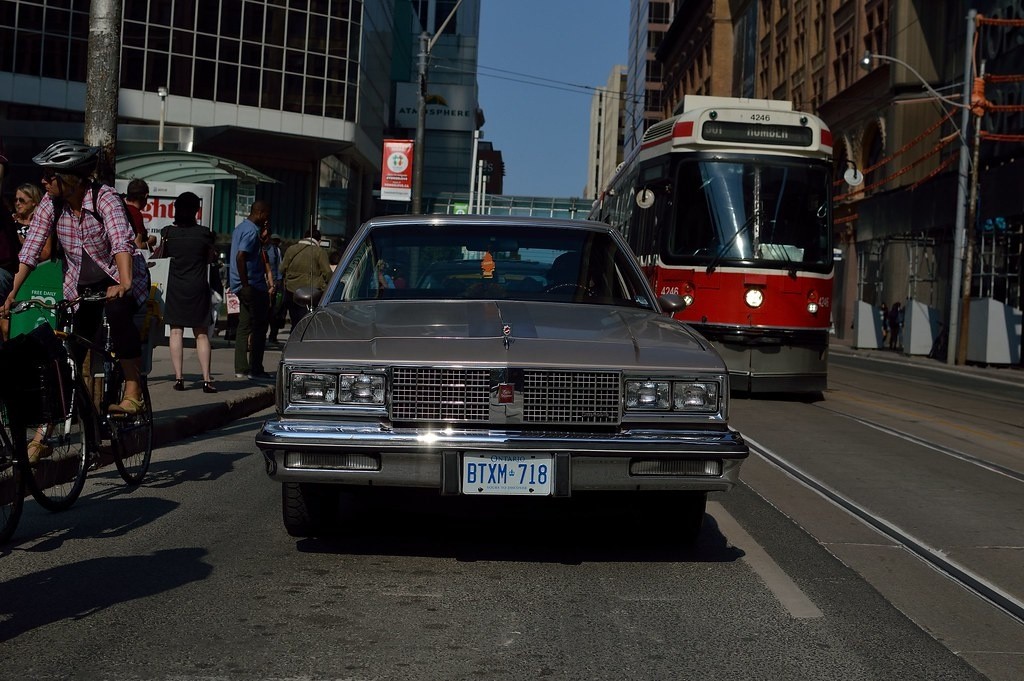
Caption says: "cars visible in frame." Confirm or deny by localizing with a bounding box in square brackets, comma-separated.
[255, 213, 750, 539]
[415, 257, 553, 293]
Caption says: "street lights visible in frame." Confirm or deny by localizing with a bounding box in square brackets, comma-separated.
[861, 52, 972, 365]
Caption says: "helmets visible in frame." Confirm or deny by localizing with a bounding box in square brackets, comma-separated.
[31, 139, 102, 169]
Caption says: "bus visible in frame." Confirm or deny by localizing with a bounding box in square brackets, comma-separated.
[587, 94, 865, 394]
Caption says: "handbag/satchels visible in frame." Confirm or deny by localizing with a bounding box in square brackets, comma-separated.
[149, 225, 169, 260]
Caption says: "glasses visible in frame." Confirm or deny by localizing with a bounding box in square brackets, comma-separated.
[15, 197, 31, 203]
[42, 170, 59, 182]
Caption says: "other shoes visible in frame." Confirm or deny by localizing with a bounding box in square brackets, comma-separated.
[203, 381, 218, 393]
[249, 374, 275, 381]
[235, 373, 249, 378]
[28, 442, 53, 463]
[109, 397, 146, 413]
[172, 379, 184, 391]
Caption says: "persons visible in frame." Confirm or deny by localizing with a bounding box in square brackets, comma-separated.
[881, 302, 904, 351]
[126, 180, 157, 248]
[0, 150, 16, 341]
[160, 192, 219, 393]
[372, 260, 406, 290]
[11, 183, 51, 260]
[281, 230, 333, 334]
[229, 200, 275, 381]
[0, 139, 150, 466]
[262, 234, 286, 344]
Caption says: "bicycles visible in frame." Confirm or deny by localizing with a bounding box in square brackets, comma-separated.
[0, 291, 154, 554]
